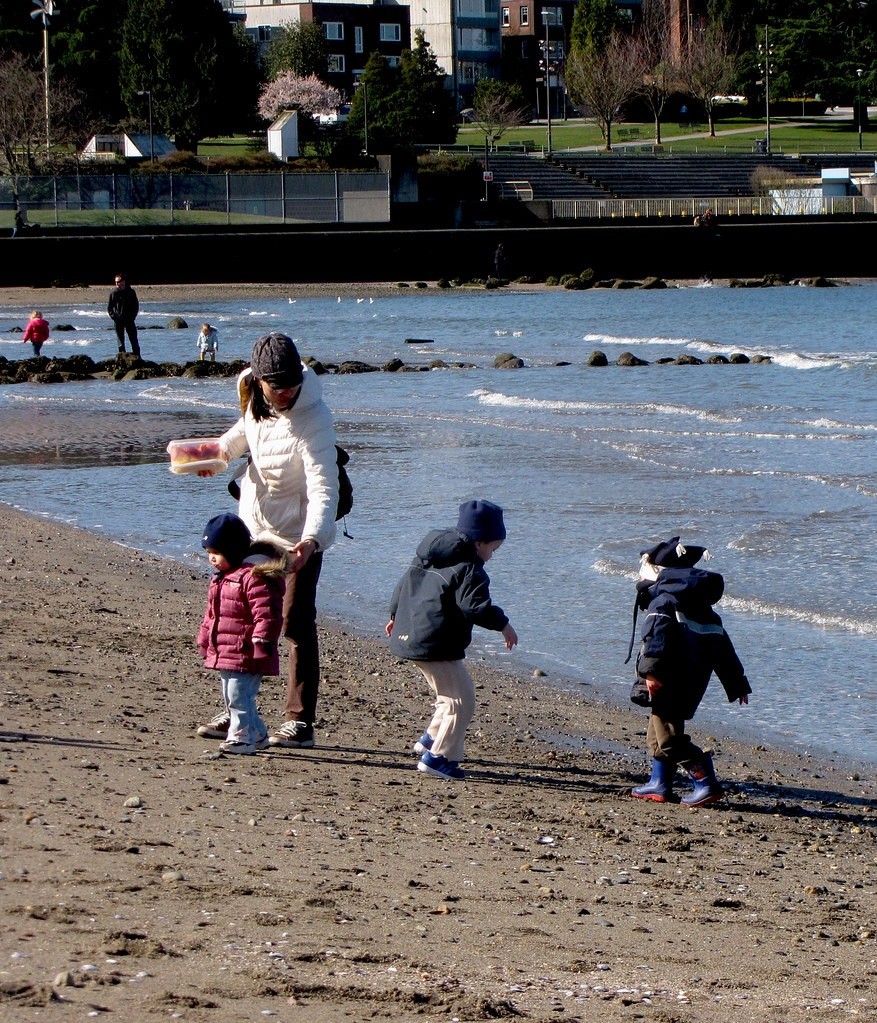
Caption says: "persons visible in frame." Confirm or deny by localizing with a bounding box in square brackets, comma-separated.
[197, 331, 340, 746]
[24, 310, 49, 356]
[11, 210, 26, 237]
[625, 535, 753, 806]
[107, 274, 140, 355]
[197, 323, 219, 361]
[385, 499, 519, 781]
[195, 512, 291, 754]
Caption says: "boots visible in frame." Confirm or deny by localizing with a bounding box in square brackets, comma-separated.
[631, 756, 678, 804]
[680, 750, 724, 807]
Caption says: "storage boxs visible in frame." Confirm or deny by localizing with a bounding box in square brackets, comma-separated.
[166, 439, 228, 475]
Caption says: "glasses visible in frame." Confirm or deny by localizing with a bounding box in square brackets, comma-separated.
[115, 279, 125, 284]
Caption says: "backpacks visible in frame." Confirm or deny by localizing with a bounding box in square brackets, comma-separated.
[227, 443, 355, 541]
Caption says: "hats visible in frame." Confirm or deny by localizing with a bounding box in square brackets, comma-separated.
[200, 511, 252, 568]
[251, 330, 304, 388]
[456, 497, 507, 542]
[637, 536, 711, 581]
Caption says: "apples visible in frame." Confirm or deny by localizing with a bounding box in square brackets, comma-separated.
[172, 445, 219, 463]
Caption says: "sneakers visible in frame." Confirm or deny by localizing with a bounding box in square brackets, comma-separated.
[267, 719, 315, 747]
[196, 711, 231, 739]
[417, 750, 464, 781]
[251, 733, 270, 751]
[412, 730, 435, 757]
[217, 740, 257, 756]
